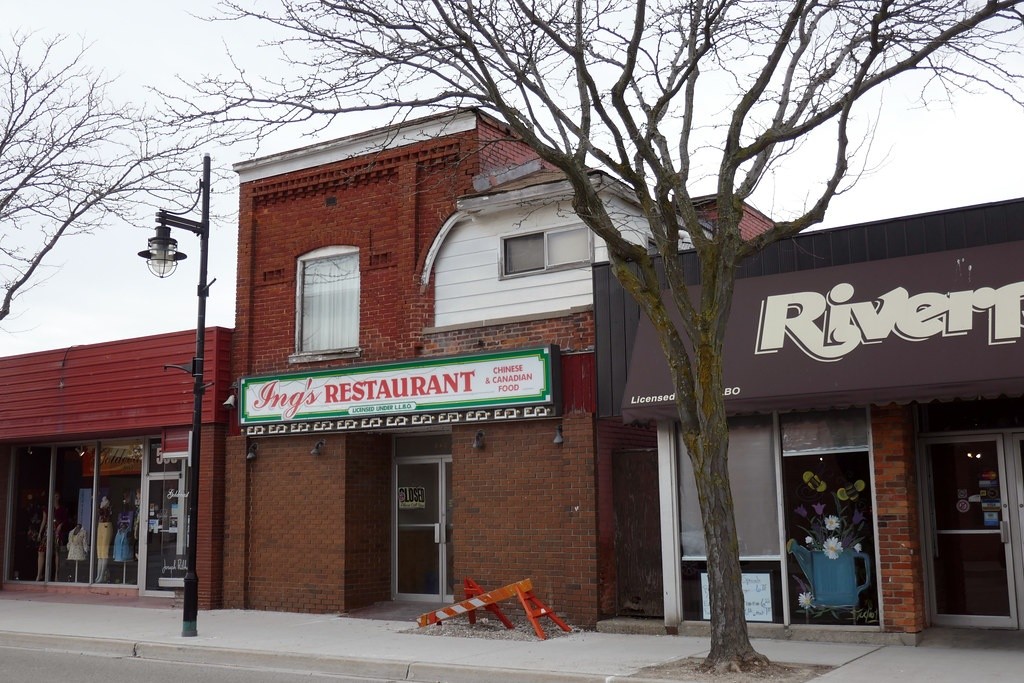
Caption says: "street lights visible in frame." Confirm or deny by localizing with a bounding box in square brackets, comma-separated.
[131, 152, 203, 639]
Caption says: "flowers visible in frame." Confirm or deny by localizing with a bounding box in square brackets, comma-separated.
[789, 501, 872, 559]
[798, 591, 816, 609]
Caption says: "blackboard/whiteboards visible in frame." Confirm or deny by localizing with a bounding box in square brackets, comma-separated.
[699, 570, 774, 624]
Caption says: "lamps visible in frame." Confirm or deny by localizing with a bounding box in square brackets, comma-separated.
[553, 424, 563, 443]
[472, 429, 486, 448]
[311, 441, 324, 454]
[246, 442, 257, 458]
